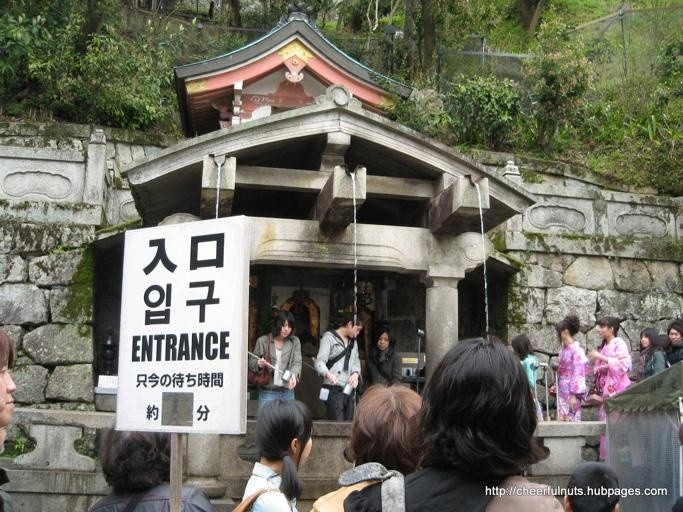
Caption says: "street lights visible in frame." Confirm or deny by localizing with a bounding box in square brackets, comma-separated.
[248, 357, 272, 387]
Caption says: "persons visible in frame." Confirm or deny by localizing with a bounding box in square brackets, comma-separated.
[313, 383, 427, 512]
[242, 399, 313, 512]
[364, 325, 402, 388]
[389, 320, 423, 352]
[0, 329, 16, 511]
[669, 423, 683, 511]
[338, 335, 564, 511]
[89, 422, 215, 512]
[562, 462, 621, 512]
[509, 316, 683, 461]
[249, 310, 302, 407]
[314, 311, 364, 420]
